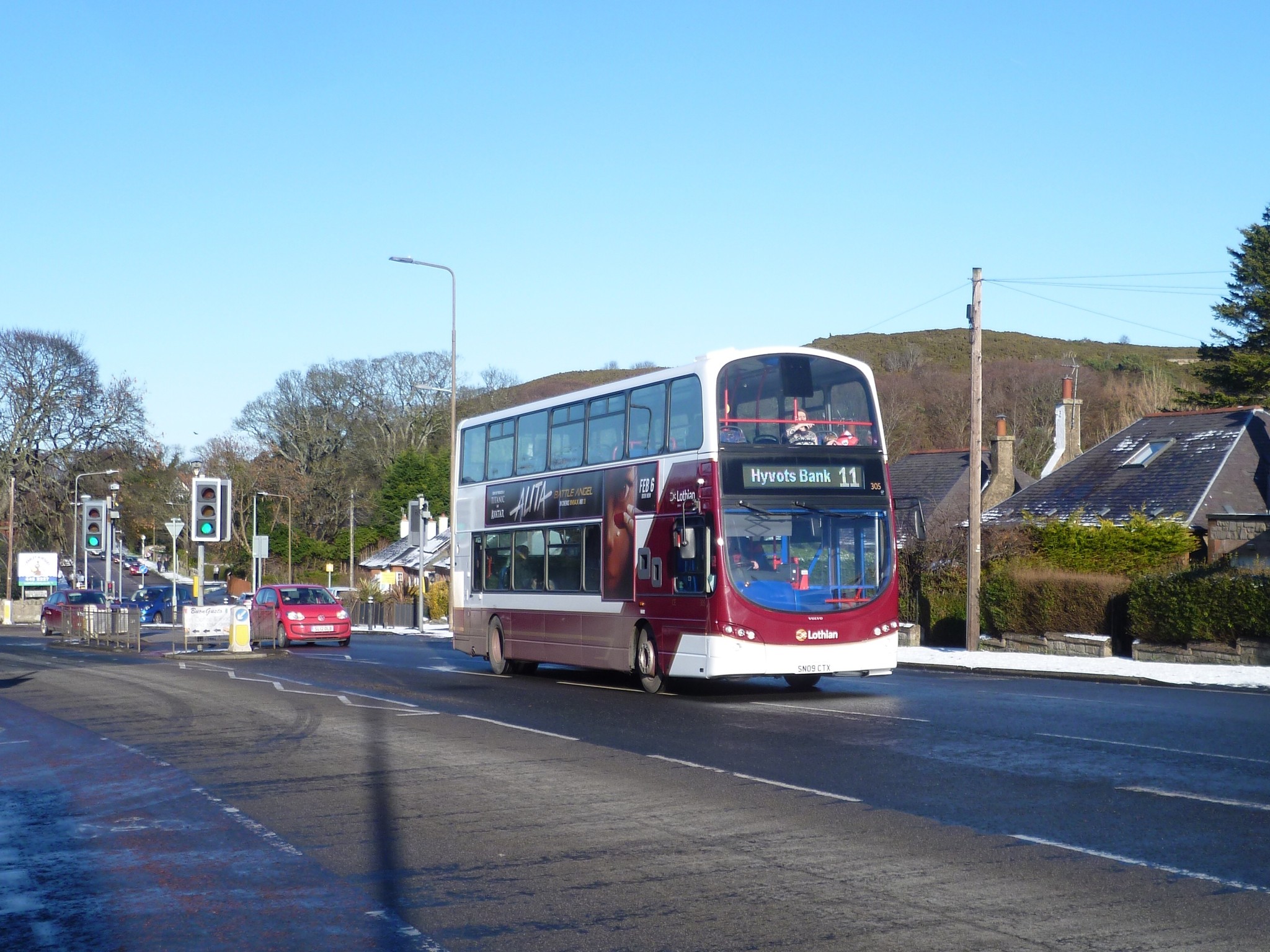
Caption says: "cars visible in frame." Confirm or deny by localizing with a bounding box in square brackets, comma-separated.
[251, 584, 351, 648]
[90, 551, 139, 570]
[120, 586, 193, 625]
[129, 562, 147, 576]
[67, 570, 91, 589]
[60, 558, 74, 567]
[40, 589, 121, 638]
[240, 592, 256, 611]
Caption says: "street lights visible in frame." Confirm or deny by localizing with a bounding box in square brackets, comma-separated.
[165, 502, 190, 577]
[72, 469, 119, 602]
[258, 492, 291, 585]
[388, 254, 457, 633]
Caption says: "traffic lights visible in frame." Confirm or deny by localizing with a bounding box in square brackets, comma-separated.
[191, 477, 221, 542]
[82, 500, 107, 556]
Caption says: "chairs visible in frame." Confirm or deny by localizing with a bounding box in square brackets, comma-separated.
[465, 427, 847, 480]
[497, 565, 580, 590]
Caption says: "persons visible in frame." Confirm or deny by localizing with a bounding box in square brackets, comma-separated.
[282, 592, 295, 604]
[147, 551, 153, 561]
[727, 538, 766, 570]
[825, 434, 837, 440]
[164, 558, 170, 572]
[784, 410, 818, 445]
[213, 565, 219, 581]
[157, 560, 162, 572]
[503, 546, 542, 590]
[605, 466, 637, 593]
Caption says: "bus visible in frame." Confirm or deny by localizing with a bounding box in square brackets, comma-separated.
[452, 344, 928, 694]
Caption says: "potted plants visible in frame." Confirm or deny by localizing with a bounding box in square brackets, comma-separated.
[348, 578, 380, 626]
[380, 576, 416, 627]
